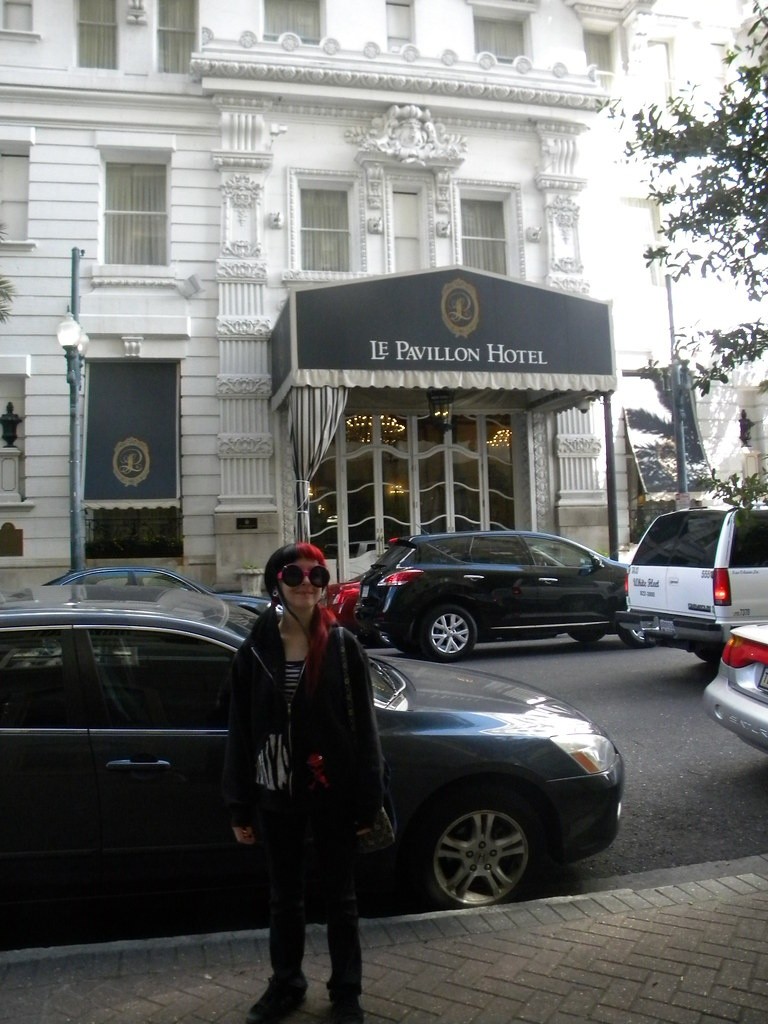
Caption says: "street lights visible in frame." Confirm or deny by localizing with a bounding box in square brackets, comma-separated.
[655, 272, 693, 510]
[57, 244, 90, 573]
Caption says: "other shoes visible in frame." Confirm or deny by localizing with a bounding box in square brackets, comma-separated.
[245, 986, 307, 1024]
[329, 995, 364, 1024]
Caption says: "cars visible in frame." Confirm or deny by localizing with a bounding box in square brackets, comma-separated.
[0, 597, 629, 913]
[317, 543, 566, 649]
[0, 564, 283, 624]
[699, 622, 768, 758]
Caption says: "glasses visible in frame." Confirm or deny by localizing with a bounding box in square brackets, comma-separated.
[275, 564, 331, 588]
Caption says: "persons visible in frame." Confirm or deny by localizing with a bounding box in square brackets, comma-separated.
[221, 542, 387, 1024]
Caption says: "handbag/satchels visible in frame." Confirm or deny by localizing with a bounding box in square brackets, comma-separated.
[348, 797, 395, 854]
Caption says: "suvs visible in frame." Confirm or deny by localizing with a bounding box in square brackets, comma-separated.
[354, 530, 662, 664]
[613, 505, 768, 663]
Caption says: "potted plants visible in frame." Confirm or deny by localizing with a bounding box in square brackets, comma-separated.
[234, 563, 264, 596]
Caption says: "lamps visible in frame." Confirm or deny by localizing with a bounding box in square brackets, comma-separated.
[428, 391, 452, 416]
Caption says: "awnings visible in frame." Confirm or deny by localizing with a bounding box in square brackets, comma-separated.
[265, 262, 616, 416]
